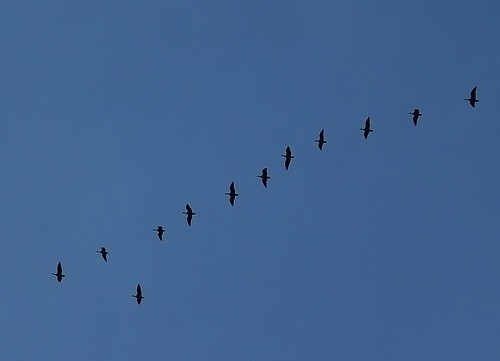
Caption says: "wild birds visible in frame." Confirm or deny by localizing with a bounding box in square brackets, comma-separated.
[359, 116, 373, 139]
[257, 167, 271, 189]
[52, 261, 65, 282]
[315, 129, 327, 150]
[153, 226, 165, 242]
[281, 146, 295, 171]
[409, 109, 422, 127]
[182, 204, 196, 225]
[464, 87, 479, 108]
[131, 283, 144, 306]
[225, 182, 238, 207]
[96, 247, 109, 263]
